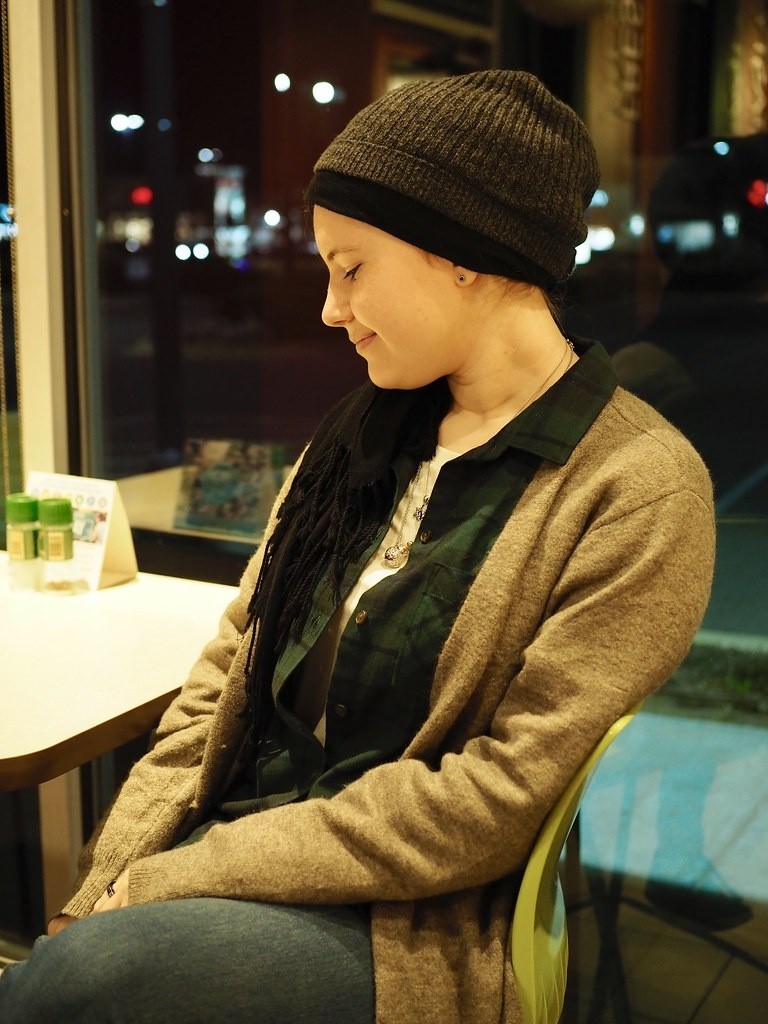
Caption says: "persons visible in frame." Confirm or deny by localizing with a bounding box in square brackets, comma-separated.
[0, 71, 713, 1023]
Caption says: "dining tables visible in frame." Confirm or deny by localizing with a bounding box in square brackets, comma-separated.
[0, 572, 243, 792]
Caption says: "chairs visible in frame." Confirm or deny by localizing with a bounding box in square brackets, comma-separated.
[510, 712, 768, 1024]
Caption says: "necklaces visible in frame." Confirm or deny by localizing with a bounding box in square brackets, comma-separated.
[385, 340, 573, 569]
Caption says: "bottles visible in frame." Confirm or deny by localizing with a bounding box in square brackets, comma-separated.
[5, 491, 43, 593]
[35, 499, 78, 591]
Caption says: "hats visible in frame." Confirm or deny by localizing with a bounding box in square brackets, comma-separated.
[305, 68, 605, 295]
